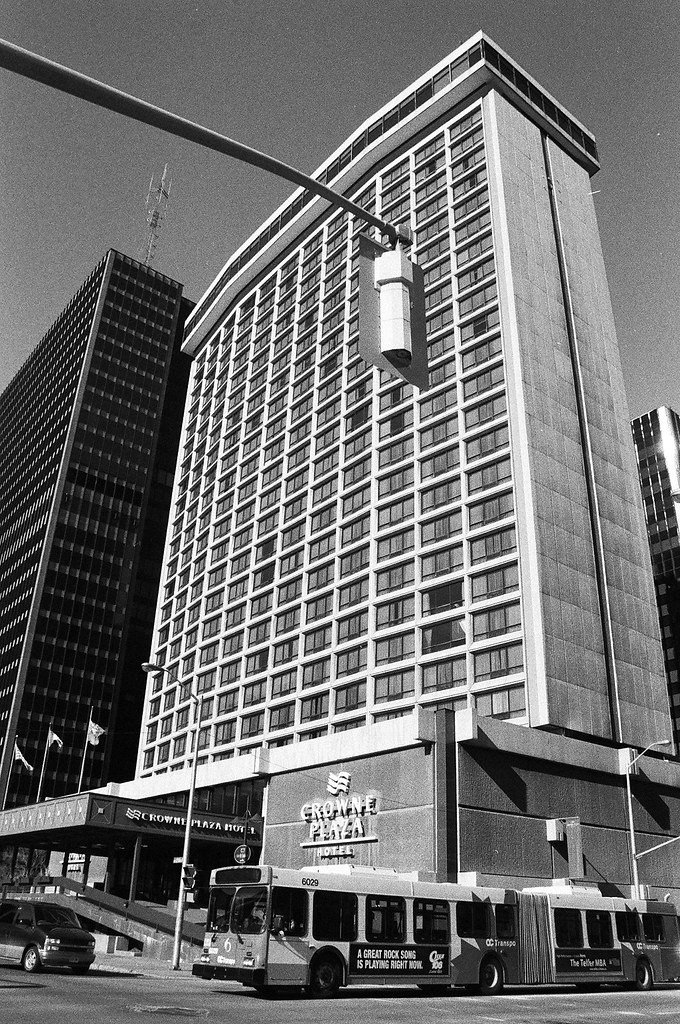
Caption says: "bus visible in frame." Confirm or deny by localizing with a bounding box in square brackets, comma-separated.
[191, 865, 680, 998]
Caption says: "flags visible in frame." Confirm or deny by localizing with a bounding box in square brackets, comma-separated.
[87, 719, 106, 745]
[47, 729, 63, 748]
[15, 742, 33, 771]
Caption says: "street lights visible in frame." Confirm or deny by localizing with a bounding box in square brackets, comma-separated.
[626, 738, 672, 944]
[141, 661, 202, 974]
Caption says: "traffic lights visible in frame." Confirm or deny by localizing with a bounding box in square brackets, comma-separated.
[182, 864, 197, 891]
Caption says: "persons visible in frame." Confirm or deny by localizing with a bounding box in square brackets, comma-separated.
[239, 917, 251, 934]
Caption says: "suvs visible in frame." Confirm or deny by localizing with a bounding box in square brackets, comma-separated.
[0, 898, 95, 973]
